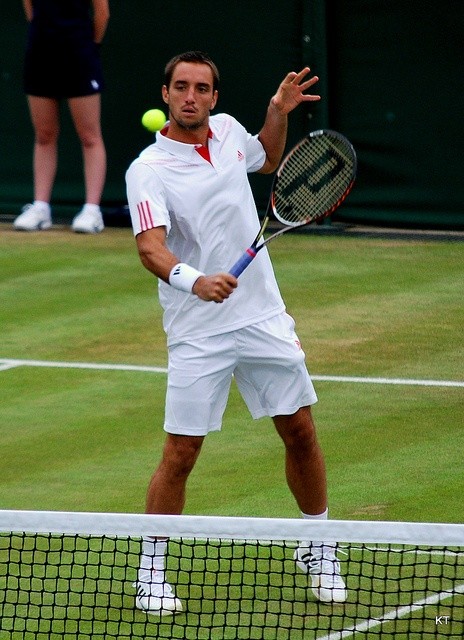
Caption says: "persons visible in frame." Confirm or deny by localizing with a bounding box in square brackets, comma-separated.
[125, 51, 348, 618]
[13, 0, 109, 233]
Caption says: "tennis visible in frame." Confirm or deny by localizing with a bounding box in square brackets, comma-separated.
[142, 108, 167, 131]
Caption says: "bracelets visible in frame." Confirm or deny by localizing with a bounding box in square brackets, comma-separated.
[170, 261, 206, 293]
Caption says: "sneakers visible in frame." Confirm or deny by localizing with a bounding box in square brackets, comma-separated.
[132, 568, 182, 616]
[14, 204, 52, 230]
[293, 541, 348, 605]
[72, 209, 105, 233]
